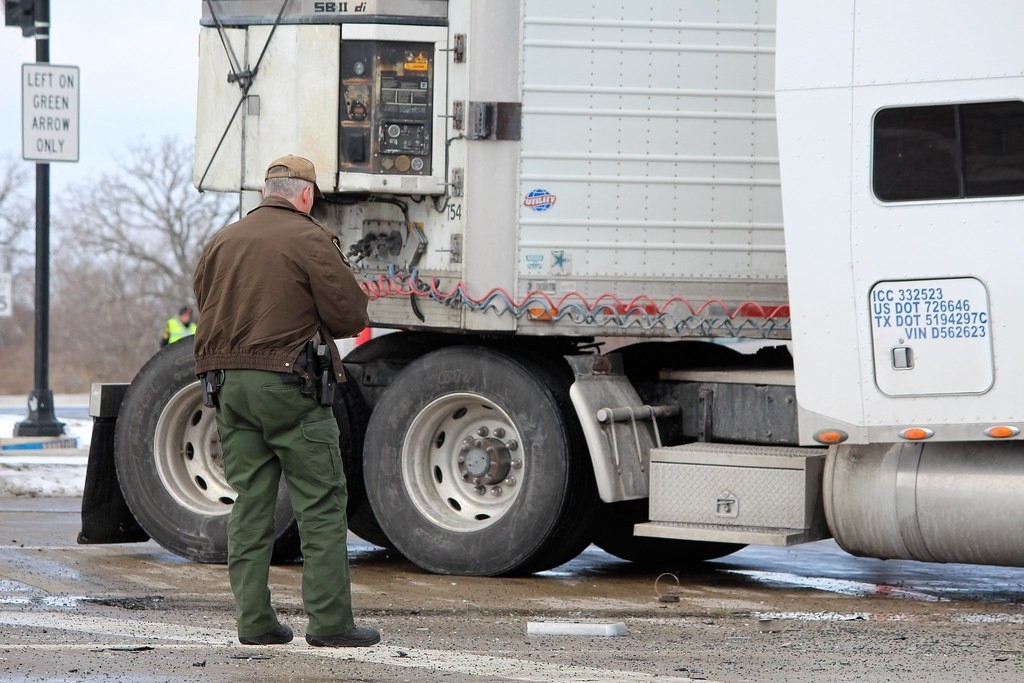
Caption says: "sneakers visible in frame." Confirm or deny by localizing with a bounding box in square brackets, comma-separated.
[304, 624, 382, 648]
[239, 622, 293, 645]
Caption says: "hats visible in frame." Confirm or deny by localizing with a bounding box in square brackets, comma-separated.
[264, 154, 325, 202]
[178, 305, 195, 315]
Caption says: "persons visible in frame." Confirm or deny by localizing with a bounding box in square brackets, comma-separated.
[159, 305, 197, 349]
[189, 153, 385, 649]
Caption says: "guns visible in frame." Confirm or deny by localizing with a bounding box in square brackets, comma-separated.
[207, 370, 225, 393]
[317, 345, 331, 375]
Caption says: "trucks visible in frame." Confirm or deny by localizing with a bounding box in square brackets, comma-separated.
[76, 0, 1022, 574]
